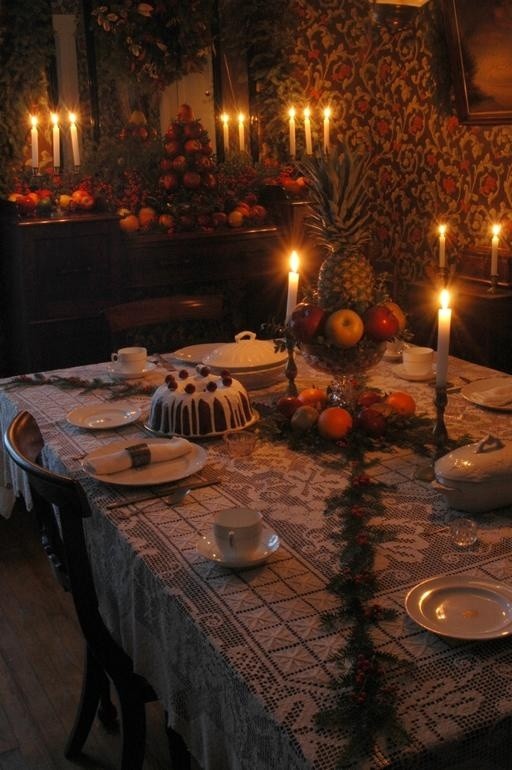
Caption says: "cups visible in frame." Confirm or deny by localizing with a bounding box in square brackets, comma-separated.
[386, 335, 402, 350]
[401, 345, 434, 376]
[112, 346, 149, 372]
[222, 429, 257, 459]
[449, 520, 478, 549]
[211, 507, 264, 560]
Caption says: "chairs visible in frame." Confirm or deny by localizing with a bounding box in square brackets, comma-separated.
[94, 284, 240, 354]
[0, 409, 195, 770]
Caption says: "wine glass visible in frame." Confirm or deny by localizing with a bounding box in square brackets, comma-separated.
[301, 342, 386, 410]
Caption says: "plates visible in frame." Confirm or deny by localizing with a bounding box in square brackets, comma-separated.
[404, 574, 512, 641]
[382, 352, 403, 361]
[392, 362, 438, 380]
[175, 341, 226, 361]
[64, 403, 142, 429]
[194, 529, 281, 569]
[460, 376, 512, 409]
[80, 439, 212, 486]
[107, 362, 155, 378]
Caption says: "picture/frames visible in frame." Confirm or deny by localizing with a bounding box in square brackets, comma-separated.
[439, 1, 512, 129]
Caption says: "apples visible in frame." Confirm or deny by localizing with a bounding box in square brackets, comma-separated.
[278, 387, 417, 441]
[8, 104, 310, 234]
[291, 291, 407, 348]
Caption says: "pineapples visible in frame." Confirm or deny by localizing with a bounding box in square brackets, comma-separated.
[317, 247, 376, 312]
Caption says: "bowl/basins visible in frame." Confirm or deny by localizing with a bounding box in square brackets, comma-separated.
[430, 435, 511, 514]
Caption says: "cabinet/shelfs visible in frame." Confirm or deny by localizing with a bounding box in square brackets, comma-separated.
[264, 198, 325, 285]
[112, 218, 290, 296]
[15, 210, 118, 373]
[406, 277, 511, 374]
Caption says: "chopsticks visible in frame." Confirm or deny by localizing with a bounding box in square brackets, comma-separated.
[106, 478, 221, 510]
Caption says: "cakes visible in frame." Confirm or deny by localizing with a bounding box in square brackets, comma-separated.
[148, 364, 254, 438]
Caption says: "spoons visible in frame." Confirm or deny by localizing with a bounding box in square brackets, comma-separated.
[457, 375, 487, 383]
[120, 486, 193, 521]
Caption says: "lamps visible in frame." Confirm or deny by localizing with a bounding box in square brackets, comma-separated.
[366, 0, 430, 36]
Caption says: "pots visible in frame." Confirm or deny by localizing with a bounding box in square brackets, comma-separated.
[203, 330, 294, 389]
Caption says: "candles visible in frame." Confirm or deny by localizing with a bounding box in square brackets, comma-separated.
[238, 112, 245, 153]
[220, 112, 230, 156]
[287, 101, 332, 162]
[27, 110, 80, 176]
[437, 221, 446, 271]
[489, 221, 500, 278]
[432, 286, 453, 389]
[282, 251, 306, 331]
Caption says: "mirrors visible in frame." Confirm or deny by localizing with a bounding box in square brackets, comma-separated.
[36, 1, 98, 169]
[154, 15, 260, 175]
